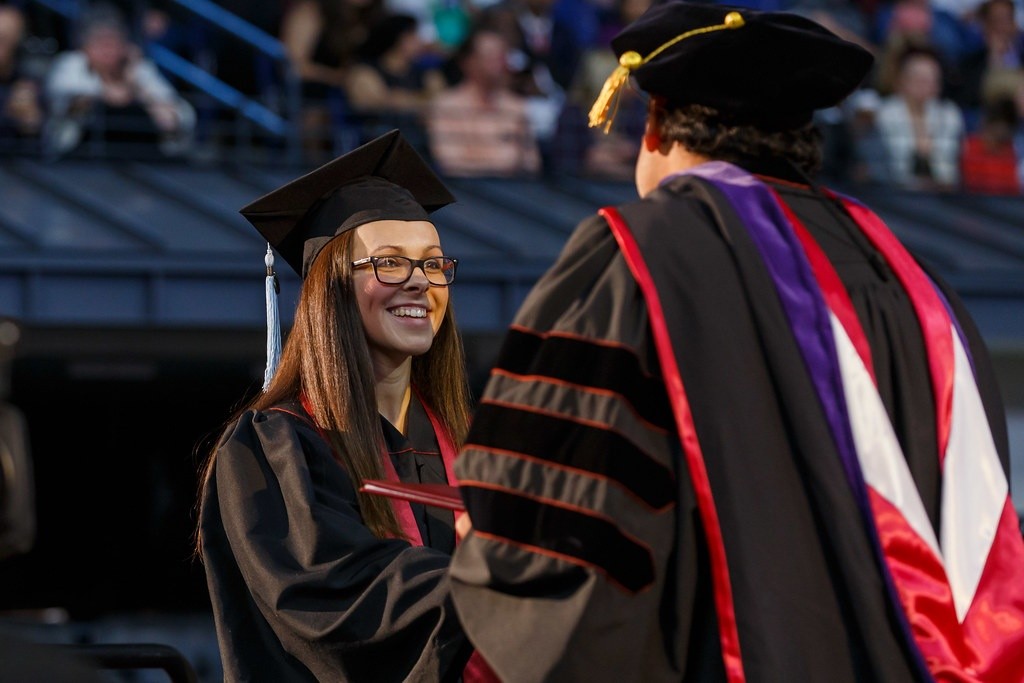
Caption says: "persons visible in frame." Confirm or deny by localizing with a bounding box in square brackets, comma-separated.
[197, 130, 474, 683]
[447, 0, 1024, 683]
[0, 0, 1024, 183]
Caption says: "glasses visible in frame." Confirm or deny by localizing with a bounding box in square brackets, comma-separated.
[352, 255, 459, 286]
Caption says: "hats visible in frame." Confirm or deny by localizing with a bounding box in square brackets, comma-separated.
[239, 128, 458, 393]
[587, 0, 875, 133]
